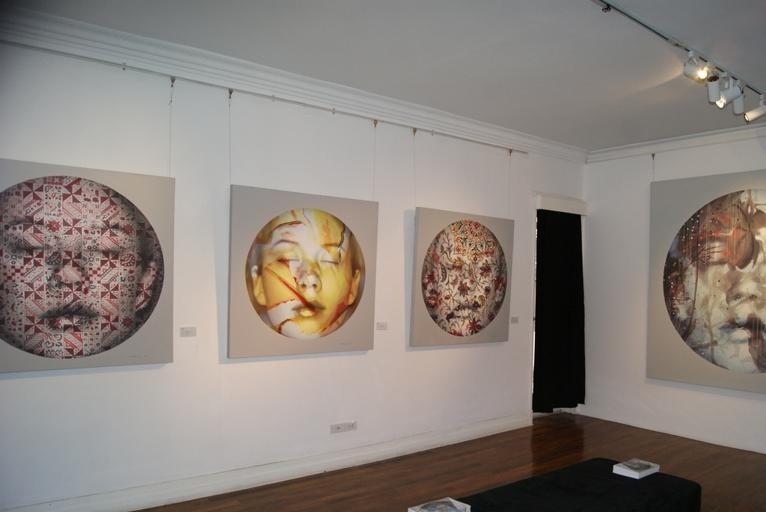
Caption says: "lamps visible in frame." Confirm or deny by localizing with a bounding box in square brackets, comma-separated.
[677, 47, 765, 141]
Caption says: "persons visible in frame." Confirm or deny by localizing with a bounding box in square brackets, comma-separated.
[251, 208, 362, 338]
[424, 221, 505, 335]
[0, 177, 156, 358]
[671, 188, 766, 374]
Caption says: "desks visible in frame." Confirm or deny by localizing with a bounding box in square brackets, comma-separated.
[457, 454, 702, 510]
